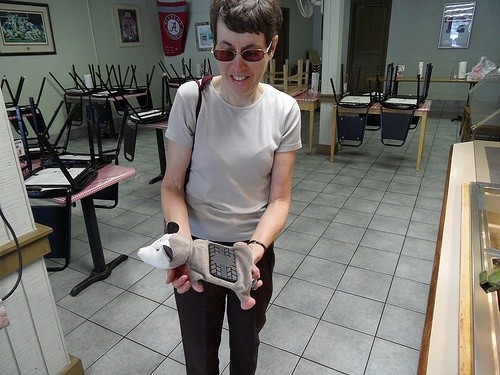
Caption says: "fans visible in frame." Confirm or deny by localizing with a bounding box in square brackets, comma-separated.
[296, 0, 324, 18]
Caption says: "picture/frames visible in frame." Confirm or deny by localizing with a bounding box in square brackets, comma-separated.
[113, 4, 143, 48]
[437, 2, 476, 49]
[0, 0, 57, 56]
[195, 22, 214, 52]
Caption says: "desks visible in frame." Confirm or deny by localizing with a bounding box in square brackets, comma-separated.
[330, 100, 432, 171]
[128, 120, 168, 185]
[22, 162, 135, 297]
[292, 89, 320, 155]
[7, 113, 32, 120]
[65, 93, 147, 140]
[391, 77, 479, 122]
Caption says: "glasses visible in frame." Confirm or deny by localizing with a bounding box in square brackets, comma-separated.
[211, 37, 274, 62]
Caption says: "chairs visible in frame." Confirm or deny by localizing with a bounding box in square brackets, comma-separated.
[329, 61, 434, 148]
[305, 49, 322, 76]
[0, 58, 311, 273]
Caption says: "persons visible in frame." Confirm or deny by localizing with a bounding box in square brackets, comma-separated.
[161, 0, 302, 375]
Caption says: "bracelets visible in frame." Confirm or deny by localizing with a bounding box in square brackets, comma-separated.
[248, 240, 266, 252]
